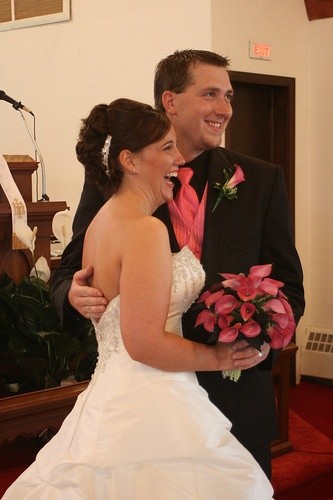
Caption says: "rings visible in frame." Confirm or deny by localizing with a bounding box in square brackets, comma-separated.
[258, 352, 263, 358]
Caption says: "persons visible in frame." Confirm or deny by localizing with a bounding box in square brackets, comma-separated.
[48, 50, 305, 479]
[0, 98, 272, 500]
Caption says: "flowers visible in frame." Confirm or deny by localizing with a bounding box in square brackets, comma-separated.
[0, 206, 96, 396]
[212, 163, 245, 212]
[192, 264, 296, 382]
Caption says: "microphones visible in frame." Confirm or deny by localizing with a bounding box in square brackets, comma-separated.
[0, 90, 34, 116]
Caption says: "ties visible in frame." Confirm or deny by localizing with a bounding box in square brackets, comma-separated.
[176, 166, 199, 228]
